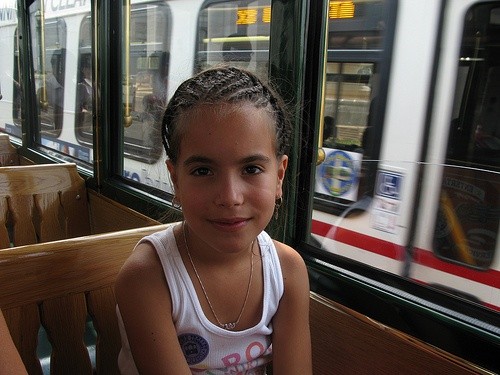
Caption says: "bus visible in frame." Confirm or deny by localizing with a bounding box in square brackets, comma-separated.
[0, 0, 500, 311]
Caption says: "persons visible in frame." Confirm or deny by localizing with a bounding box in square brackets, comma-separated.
[75, 53, 92, 138]
[50, 48, 66, 134]
[131, 50, 170, 153]
[222, 32, 254, 68]
[322, 116, 360, 151]
[113, 66, 314, 375]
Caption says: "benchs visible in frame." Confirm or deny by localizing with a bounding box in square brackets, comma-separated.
[0, 161, 91, 250]
[0, 221, 184, 375]
[0, 133, 19, 167]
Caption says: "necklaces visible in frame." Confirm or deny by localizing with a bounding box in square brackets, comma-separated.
[182, 220, 255, 329]
